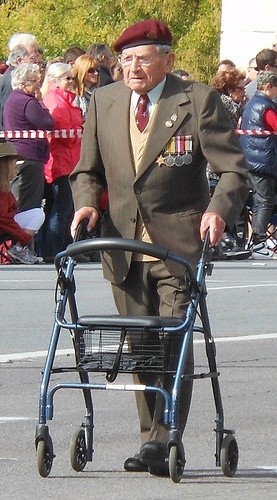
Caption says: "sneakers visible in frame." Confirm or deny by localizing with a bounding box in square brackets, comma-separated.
[221, 241, 252, 261]
[8, 245, 44, 265]
[246, 238, 277, 260]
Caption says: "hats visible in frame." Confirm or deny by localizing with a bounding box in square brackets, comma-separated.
[0, 141, 18, 158]
[112, 20, 173, 52]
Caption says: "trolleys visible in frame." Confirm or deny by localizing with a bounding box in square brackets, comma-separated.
[32, 220, 239, 484]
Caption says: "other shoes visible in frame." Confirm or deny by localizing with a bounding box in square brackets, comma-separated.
[123, 452, 148, 472]
[140, 444, 172, 475]
[42, 254, 90, 266]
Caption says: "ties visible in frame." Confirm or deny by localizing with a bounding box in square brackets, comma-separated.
[134, 94, 150, 133]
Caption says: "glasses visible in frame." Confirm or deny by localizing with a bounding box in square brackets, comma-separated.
[235, 86, 246, 92]
[33, 62, 44, 66]
[59, 76, 75, 81]
[249, 67, 259, 71]
[20, 53, 40, 61]
[27, 79, 42, 84]
[117, 51, 169, 67]
[87, 67, 99, 73]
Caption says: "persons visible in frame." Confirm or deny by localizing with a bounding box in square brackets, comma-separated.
[42, 63, 84, 264]
[240, 72, 277, 259]
[0, 34, 124, 264]
[173, 49, 277, 259]
[4, 63, 54, 264]
[71, 19, 249, 478]
